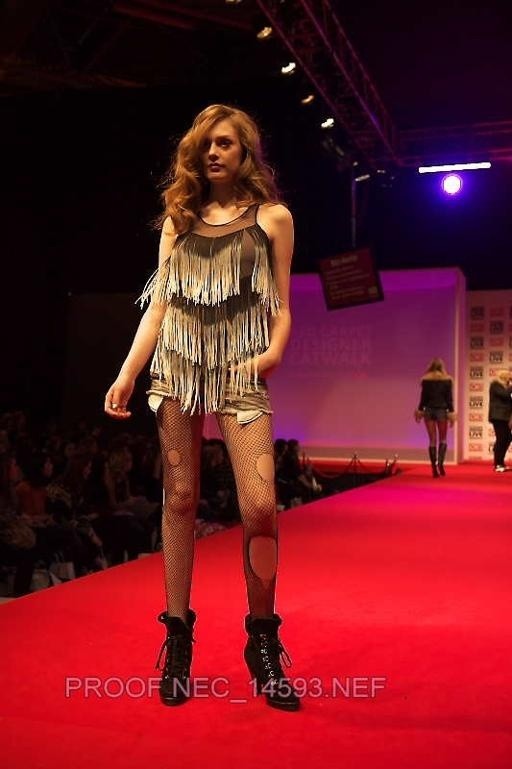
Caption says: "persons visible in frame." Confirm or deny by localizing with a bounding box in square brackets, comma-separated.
[105, 104, 299, 711]
[0, 403, 338, 594]
[489, 368, 512, 472]
[414, 355, 454, 478]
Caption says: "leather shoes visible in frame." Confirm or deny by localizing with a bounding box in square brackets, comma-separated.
[494, 464, 512, 471]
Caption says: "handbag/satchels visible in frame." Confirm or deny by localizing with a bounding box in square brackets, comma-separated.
[47, 551, 77, 580]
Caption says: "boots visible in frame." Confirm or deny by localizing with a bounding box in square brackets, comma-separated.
[158, 609, 196, 705]
[429, 446, 438, 476]
[244, 614, 298, 709]
[438, 443, 446, 476]
